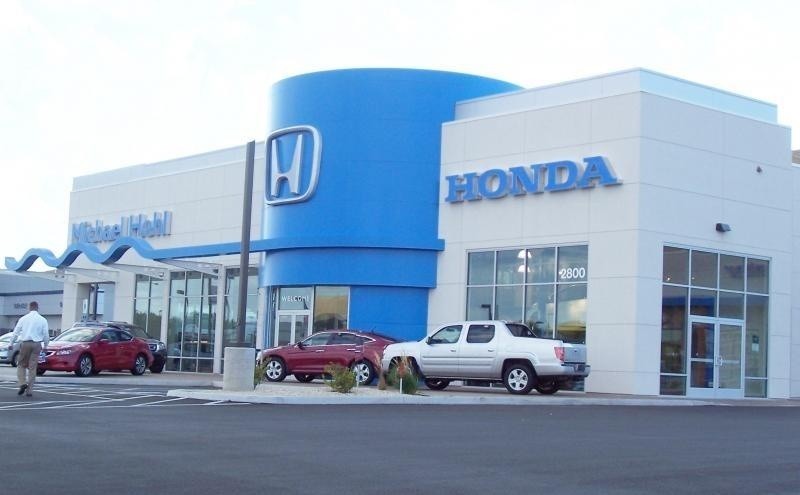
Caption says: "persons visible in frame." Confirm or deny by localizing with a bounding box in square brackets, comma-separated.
[173, 345, 180, 369]
[8, 301, 49, 397]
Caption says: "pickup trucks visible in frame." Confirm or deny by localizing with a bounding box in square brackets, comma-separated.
[380, 319, 591, 395]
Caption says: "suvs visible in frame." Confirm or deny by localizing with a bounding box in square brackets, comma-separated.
[72, 321, 166, 373]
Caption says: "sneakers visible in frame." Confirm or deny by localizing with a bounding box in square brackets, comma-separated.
[19, 384, 27, 395]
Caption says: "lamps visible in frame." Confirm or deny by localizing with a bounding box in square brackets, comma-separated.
[518, 250, 532, 272]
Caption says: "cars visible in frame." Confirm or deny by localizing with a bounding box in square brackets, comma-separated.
[0, 332, 53, 367]
[36, 326, 154, 377]
[255, 329, 398, 385]
